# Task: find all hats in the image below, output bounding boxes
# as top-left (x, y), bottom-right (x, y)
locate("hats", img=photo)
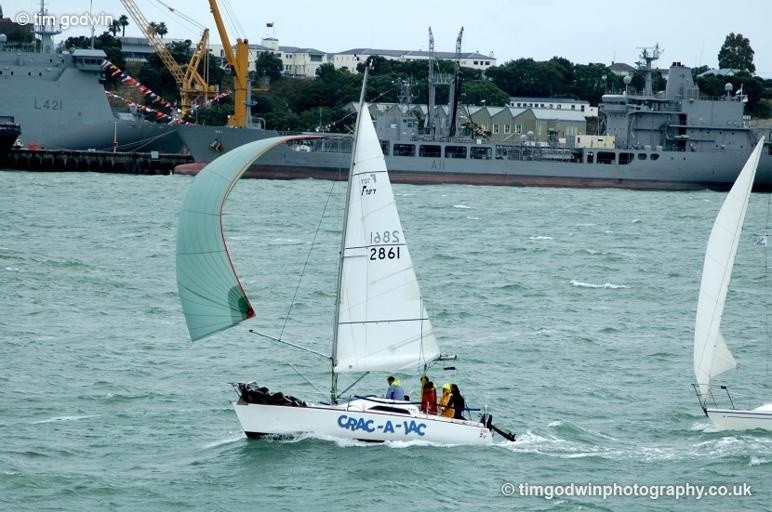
top-left (442, 384), bottom-right (450, 390)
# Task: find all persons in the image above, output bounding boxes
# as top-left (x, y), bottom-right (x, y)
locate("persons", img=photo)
top-left (419, 376), bottom-right (438, 415)
top-left (442, 384), bottom-right (464, 419)
top-left (386, 376), bottom-right (405, 400)
top-left (439, 382), bottom-right (455, 418)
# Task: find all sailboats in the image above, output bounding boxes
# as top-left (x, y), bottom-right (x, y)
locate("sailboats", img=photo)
top-left (173, 66), bottom-right (516, 449)
top-left (688, 137), bottom-right (771, 434)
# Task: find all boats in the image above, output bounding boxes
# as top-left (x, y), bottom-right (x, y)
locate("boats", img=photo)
top-left (172, 25), bottom-right (772, 195)
top-left (0, 0), bottom-right (191, 155)
